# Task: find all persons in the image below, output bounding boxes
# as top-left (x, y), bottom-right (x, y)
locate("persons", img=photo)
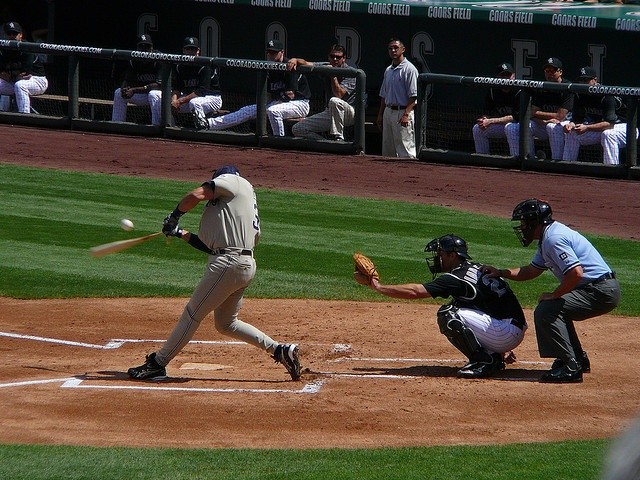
top-left (479, 198), bottom-right (620, 384)
top-left (529, 58), bottom-right (574, 161)
top-left (473, 63), bottom-right (521, 157)
top-left (192, 40), bottom-right (312, 136)
top-left (378, 38), bottom-right (419, 159)
top-left (562, 66), bottom-right (639, 165)
top-left (170, 37), bottom-right (223, 131)
top-left (112, 34), bottom-right (163, 127)
top-left (353, 234), bottom-right (528, 379)
top-left (292, 45), bottom-right (356, 141)
top-left (1, 23), bottom-right (49, 114)
top-left (129, 165), bottom-right (302, 381)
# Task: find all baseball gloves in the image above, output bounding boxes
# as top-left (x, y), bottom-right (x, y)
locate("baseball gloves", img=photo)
top-left (352, 252), bottom-right (379, 286)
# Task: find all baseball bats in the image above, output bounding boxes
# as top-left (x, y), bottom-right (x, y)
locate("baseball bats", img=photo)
top-left (88, 230), bottom-right (162, 256)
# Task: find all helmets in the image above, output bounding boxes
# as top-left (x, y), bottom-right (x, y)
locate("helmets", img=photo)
top-left (425, 234), bottom-right (472, 274)
top-left (211, 164), bottom-right (241, 178)
top-left (511, 198), bottom-right (552, 247)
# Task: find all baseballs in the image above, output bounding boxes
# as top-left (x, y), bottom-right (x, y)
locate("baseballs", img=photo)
top-left (120, 218), bottom-right (134, 232)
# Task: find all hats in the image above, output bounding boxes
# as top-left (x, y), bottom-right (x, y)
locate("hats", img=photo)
top-left (499, 63), bottom-right (516, 76)
top-left (266, 40), bottom-right (284, 52)
top-left (542, 57), bottom-right (563, 69)
top-left (184, 37), bottom-right (200, 49)
top-left (5, 22), bottom-right (22, 33)
top-left (577, 66), bottom-right (597, 79)
top-left (136, 33), bottom-right (153, 46)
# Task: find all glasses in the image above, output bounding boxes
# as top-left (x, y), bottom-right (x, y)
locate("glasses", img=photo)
top-left (329, 54), bottom-right (344, 60)
top-left (387, 45), bottom-right (402, 50)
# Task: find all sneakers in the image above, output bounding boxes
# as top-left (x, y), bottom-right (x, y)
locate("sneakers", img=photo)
top-left (191, 108), bottom-right (207, 132)
top-left (539, 360), bottom-right (584, 383)
top-left (271, 344), bottom-right (301, 382)
top-left (127, 352), bottom-right (167, 380)
top-left (578, 356), bottom-right (589, 372)
top-left (453, 355), bottom-right (498, 378)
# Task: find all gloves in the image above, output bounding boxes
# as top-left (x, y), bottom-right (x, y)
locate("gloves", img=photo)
top-left (169, 225), bottom-right (183, 240)
top-left (161, 210), bottom-right (183, 234)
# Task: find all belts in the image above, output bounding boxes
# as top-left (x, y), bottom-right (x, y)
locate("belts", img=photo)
top-left (591, 272), bottom-right (615, 283)
top-left (210, 249), bottom-right (254, 256)
top-left (500, 318), bottom-right (523, 331)
top-left (388, 104), bottom-right (408, 109)
